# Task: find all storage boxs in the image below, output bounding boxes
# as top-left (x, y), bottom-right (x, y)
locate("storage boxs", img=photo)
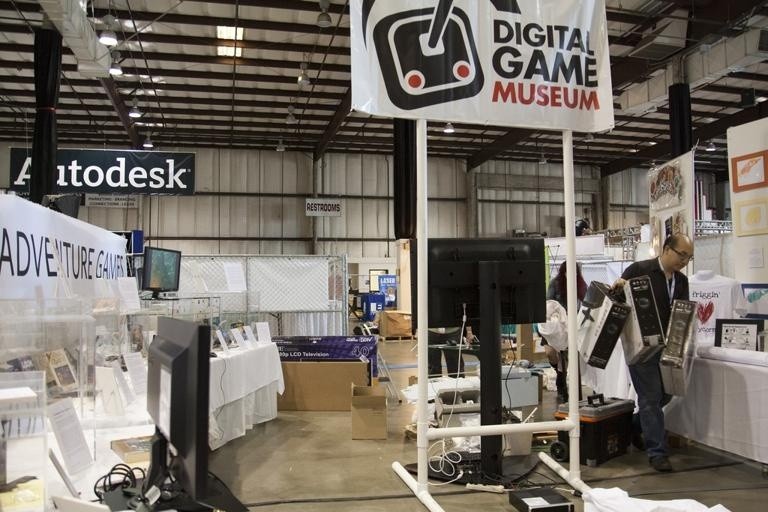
top-left (549, 392), bottom-right (636, 468)
top-left (350, 381), bottom-right (389, 441)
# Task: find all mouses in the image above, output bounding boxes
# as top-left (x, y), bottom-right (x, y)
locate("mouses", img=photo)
top-left (446, 339), bottom-right (457, 346)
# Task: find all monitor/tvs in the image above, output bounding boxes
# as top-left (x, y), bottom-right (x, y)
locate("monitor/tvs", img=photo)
top-left (126, 317), bottom-right (212, 510)
top-left (142, 247), bottom-right (181, 300)
top-left (410, 238), bottom-right (546, 328)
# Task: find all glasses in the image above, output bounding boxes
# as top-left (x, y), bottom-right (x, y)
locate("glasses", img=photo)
top-left (669, 246), bottom-right (694, 261)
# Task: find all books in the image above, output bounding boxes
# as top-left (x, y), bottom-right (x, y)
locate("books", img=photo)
top-left (109, 435), bottom-right (158, 464)
top-left (36, 347), bottom-right (79, 396)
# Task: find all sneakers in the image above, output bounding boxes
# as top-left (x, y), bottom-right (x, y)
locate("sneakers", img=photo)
top-left (631, 413), bottom-right (643, 449)
top-left (559, 394), bottom-right (568, 403)
top-left (649, 455), bottom-right (672, 471)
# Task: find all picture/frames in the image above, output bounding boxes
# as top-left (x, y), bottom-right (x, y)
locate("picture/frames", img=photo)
top-left (730, 149), bottom-right (766, 238)
top-left (713, 283), bottom-right (767, 351)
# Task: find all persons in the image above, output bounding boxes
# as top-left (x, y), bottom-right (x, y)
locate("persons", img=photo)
top-left (687, 268), bottom-right (749, 346)
top-left (540, 260), bottom-right (588, 403)
top-left (613, 232), bottom-right (695, 471)
top-left (426, 326), bottom-right (475, 377)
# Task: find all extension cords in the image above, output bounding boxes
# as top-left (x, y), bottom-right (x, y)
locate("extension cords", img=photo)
top-left (466, 483), bottom-right (505, 493)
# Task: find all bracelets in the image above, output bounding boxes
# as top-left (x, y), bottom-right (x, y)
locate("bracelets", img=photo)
top-left (466, 331), bottom-right (473, 333)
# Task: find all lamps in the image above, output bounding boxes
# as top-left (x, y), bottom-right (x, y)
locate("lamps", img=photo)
top-left (704, 137), bottom-right (716, 153)
top-left (442, 122), bottom-right (454, 134)
top-left (274, 0), bottom-right (333, 152)
top-left (97, 0), bottom-right (154, 149)
